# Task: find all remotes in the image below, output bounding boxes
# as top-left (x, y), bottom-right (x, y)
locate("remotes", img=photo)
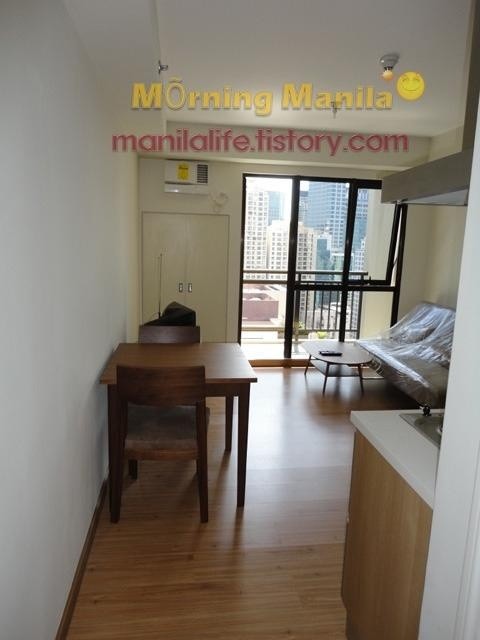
top-left (320, 350), bottom-right (342, 356)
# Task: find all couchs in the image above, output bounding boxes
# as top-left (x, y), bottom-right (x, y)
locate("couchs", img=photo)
top-left (353, 302), bottom-right (456, 409)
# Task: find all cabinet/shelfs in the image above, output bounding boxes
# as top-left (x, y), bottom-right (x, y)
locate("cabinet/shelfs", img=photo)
top-left (341, 429), bottom-right (433, 640)
top-left (141, 211), bottom-right (230, 343)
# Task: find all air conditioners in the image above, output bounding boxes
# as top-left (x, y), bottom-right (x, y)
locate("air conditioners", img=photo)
top-left (164, 160), bottom-right (209, 196)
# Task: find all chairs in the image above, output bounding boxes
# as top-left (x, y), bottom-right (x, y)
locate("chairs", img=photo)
top-left (138, 325), bottom-right (200, 344)
top-left (107, 364), bottom-right (210, 523)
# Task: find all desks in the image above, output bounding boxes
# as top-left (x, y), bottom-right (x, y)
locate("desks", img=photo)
top-left (100, 343), bottom-right (257, 507)
